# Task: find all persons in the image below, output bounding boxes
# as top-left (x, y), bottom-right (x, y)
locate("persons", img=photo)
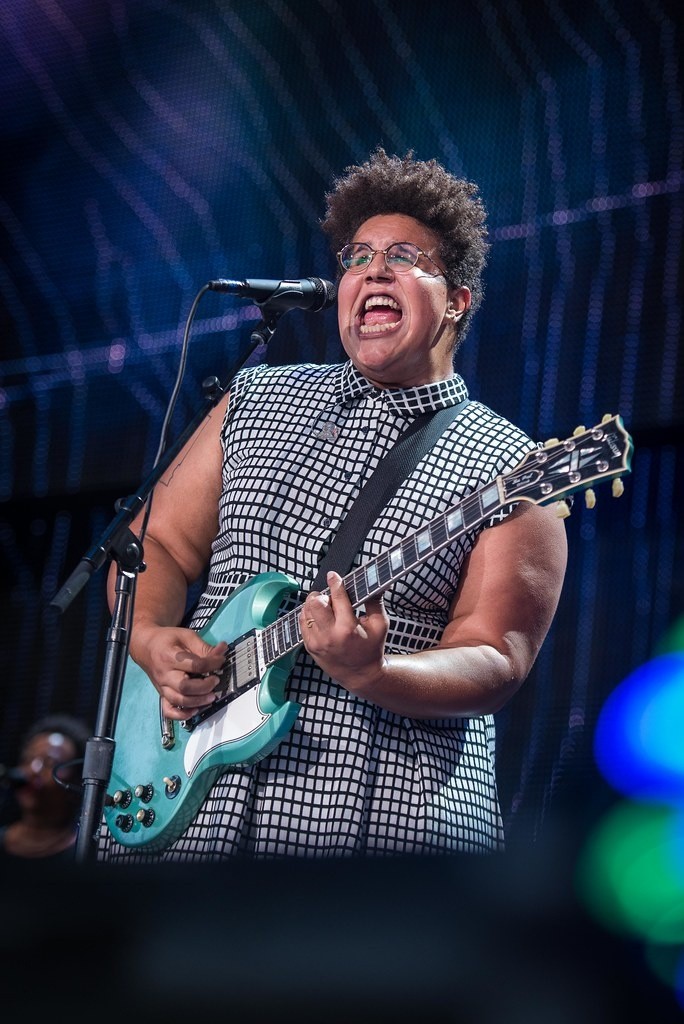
top-left (97, 153), bottom-right (566, 867)
top-left (0, 717), bottom-right (94, 876)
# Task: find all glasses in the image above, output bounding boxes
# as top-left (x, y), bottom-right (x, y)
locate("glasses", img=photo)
top-left (337, 240), bottom-right (456, 286)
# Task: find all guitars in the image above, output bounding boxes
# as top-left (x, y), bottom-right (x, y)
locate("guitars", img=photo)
top-left (97, 410), bottom-right (638, 859)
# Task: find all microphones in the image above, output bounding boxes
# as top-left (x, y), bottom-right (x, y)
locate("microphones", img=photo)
top-left (0, 768), bottom-right (28, 789)
top-left (207, 276), bottom-right (337, 313)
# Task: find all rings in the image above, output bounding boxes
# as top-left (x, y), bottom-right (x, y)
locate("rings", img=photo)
top-left (308, 619), bottom-right (315, 627)
